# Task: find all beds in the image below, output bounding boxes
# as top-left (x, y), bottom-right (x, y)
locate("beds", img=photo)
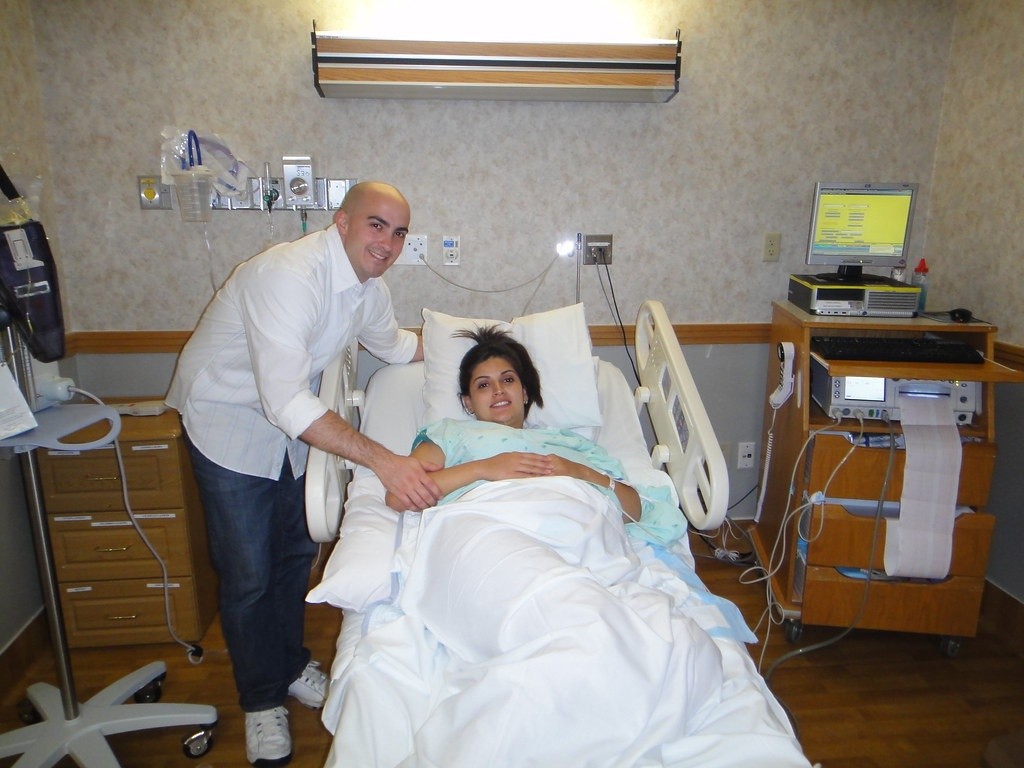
top-left (304, 300), bottom-right (814, 767)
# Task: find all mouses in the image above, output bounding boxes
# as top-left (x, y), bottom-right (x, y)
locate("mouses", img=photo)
top-left (950, 308), bottom-right (972, 324)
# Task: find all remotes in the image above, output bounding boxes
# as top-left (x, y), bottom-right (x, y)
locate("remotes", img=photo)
top-left (110, 400), bottom-right (168, 416)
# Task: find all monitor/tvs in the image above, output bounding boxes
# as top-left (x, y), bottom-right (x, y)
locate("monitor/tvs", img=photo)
top-left (804, 182), bottom-right (920, 281)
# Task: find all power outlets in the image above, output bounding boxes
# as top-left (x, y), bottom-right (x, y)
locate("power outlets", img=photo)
top-left (738, 442), bottom-right (754, 468)
top-left (395, 234), bottom-right (428, 266)
top-left (585, 234), bottom-right (612, 264)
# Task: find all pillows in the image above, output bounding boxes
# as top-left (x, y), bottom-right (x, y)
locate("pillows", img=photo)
top-left (417, 304), bottom-right (602, 428)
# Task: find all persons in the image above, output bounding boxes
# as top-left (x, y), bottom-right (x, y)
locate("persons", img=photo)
top-left (387, 327), bottom-right (642, 524)
top-left (170, 183), bottom-right (425, 760)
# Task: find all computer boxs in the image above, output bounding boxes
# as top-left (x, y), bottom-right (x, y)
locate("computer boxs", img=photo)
top-left (787, 275), bottom-right (921, 318)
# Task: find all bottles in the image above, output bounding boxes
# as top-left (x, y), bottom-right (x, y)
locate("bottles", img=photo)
top-left (911, 258), bottom-right (929, 312)
top-left (891, 266), bottom-right (906, 284)
top-left (172, 171), bottom-right (213, 223)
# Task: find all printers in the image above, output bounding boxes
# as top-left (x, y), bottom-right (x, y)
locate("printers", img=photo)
top-left (808, 358), bottom-right (982, 428)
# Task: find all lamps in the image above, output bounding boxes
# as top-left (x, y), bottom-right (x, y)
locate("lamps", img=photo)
top-left (309, 20), bottom-right (681, 102)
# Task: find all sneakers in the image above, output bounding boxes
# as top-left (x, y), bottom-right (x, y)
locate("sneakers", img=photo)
top-left (243, 705), bottom-right (292, 768)
top-left (286, 661), bottom-right (332, 710)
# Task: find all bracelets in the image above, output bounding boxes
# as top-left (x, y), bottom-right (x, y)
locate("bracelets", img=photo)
top-left (604, 474), bottom-right (615, 491)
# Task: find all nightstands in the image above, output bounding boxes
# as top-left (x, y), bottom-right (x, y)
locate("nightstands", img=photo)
top-left (37, 395), bottom-right (214, 644)
top-left (751, 302), bottom-right (1023, 639)
